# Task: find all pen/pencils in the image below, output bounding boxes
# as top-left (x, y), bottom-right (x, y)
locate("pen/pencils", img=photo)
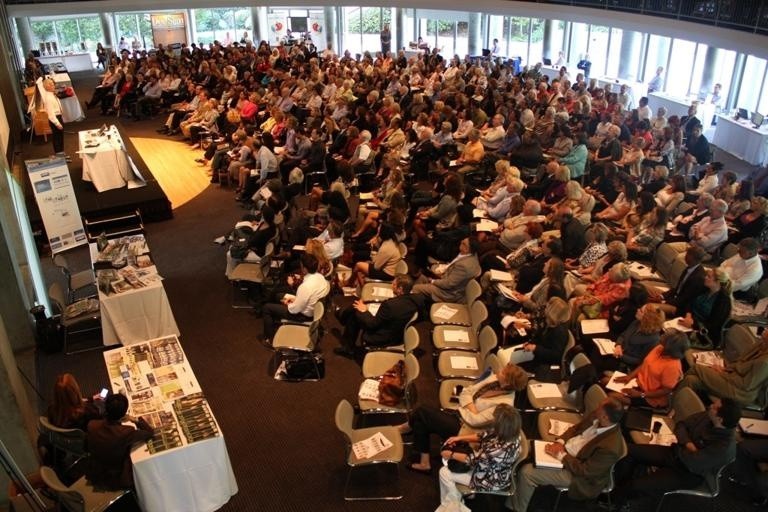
top-left (520, 307), bottom-right (523, 313)
top-left (525, 338), bottom-right (532, 346)
top-left (746, 424), bottom-right (754, 428)
top-left (555, 438), bottom-right (557, 443)
top-left (379, 438), bottom-right (384, 446)
top-left (616, 349), bottom-right (625, 358)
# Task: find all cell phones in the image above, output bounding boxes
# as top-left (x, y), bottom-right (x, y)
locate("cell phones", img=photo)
top-left (99, 387), bottom-right (109, 399)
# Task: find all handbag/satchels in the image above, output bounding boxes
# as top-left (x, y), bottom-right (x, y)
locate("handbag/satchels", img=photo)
top-left (378, 359), bottom-right (408, 407)
top-left (447, 441), bottom-right (474, 473)
top-left (267, 347), bottom-right (325, 382)
top-left (230, 234), bottom-right (250, 259)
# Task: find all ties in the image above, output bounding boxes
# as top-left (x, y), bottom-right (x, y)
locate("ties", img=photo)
top-left (54, 93), bottom-right (63, 114)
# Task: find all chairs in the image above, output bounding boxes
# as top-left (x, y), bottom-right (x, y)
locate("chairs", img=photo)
top-left (229, 195), bottom-right (768, 512)
top-left (49, 284), bottom-right (107, 357)
top-left (39, 415), bottom-right (94, 470)
top-left (52, 253), bottom-right (99, 300)
top-left (39, 464), bottom-right (138, 512)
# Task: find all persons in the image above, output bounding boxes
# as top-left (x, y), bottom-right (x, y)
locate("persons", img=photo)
top-left (43, 79), bottom-right (71, 159)
top-left (26, 53), bottom-right (45, 75)
top-left (47, 373), bottom-right (105, 453)
top-left (24, 61), bottom-right (41, 82)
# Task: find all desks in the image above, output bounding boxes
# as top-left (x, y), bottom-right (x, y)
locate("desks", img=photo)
top-left (87, 232), bottom-right (180, 348)
top-left (39, 62), bottom-right (80, 126)
top-left (102, 334), bottom-right (241, 512)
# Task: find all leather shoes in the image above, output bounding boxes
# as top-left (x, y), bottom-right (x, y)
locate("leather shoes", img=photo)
top-left (334, 306), bottom-right (345, 326)
top-left (333, 347), bottom-right (356, 361)
top-left (406, 462), bottom-right (432, 474)
top-left (65, 155), bottom-right (73, 163)
top-left (400, 429), bottom-right (413, 435)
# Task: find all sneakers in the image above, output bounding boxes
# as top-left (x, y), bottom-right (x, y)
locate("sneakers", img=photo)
top-left (84, 100), bottom-right (168, 121)
top-left (260, 339), bottom-right (273, 347)
top-left (156, 126), bottom-right (252, 210)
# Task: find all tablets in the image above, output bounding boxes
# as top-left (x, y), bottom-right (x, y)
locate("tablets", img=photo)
top-left (98, 124), bottom-right (105, 136)
